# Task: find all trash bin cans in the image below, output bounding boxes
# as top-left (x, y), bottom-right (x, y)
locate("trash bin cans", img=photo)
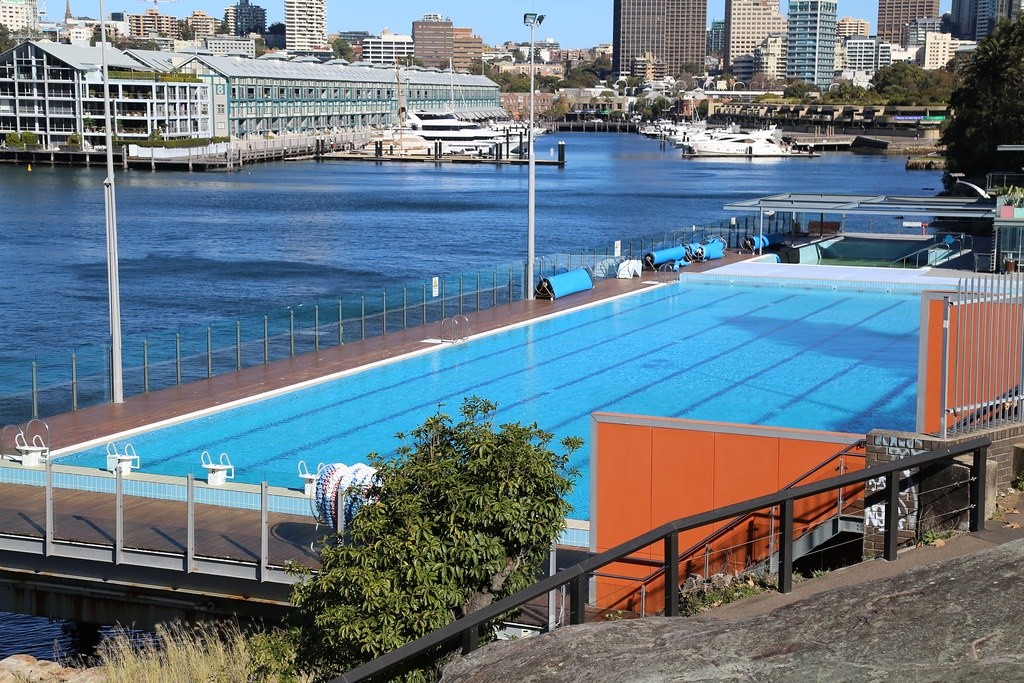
top-left (1005, 259), bottom-right (1015, 274)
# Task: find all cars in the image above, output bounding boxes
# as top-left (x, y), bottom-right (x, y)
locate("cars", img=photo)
top-left (593, 119), bottom-right (603, 123)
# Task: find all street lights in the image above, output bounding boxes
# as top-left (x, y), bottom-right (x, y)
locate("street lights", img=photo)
top-left (523, 12), bottom-right (548, 299)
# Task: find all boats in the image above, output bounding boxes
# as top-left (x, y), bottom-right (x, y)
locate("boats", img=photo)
top-left (667, 129), bottom-right (750, 147)
top-left (681, 133), bottom-right (821, 158)
top-left (508, 123), bottom-right (547, 134)
top-left (364, 127), bottom-right (451, 156)
top-left (640, 126), bottom-right (684, 136)
top-left (383, 47), bottom-right (538, 157)
